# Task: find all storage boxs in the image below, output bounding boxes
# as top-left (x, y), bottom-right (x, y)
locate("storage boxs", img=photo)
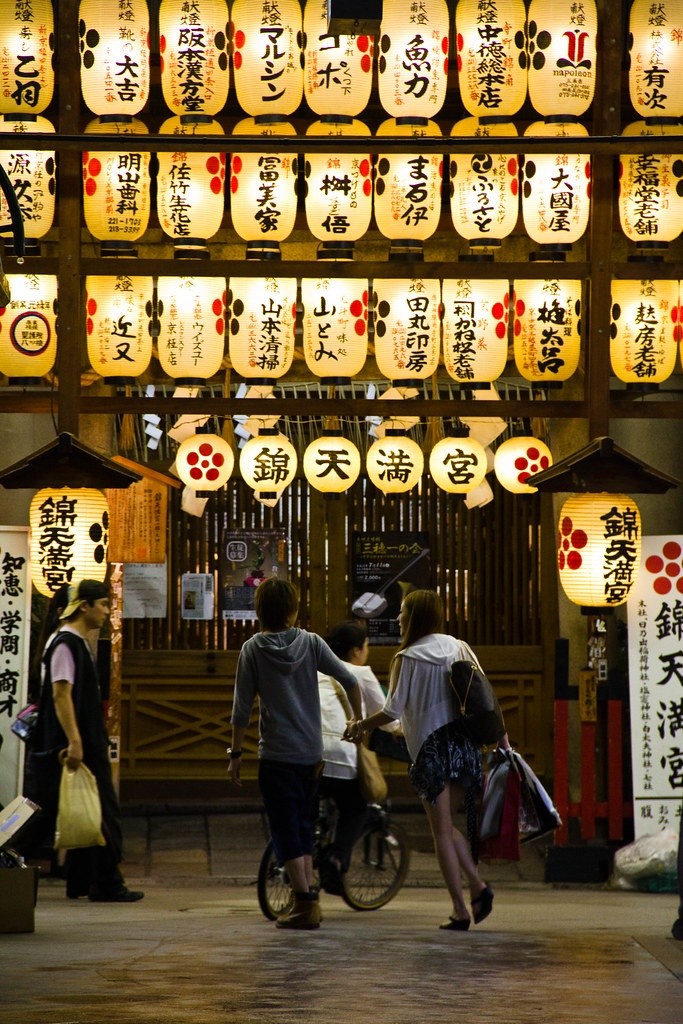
top-left (0, 795), bottom-right (42, 934)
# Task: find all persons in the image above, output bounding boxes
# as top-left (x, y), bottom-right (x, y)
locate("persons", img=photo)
top-left (9, 578), bottom-right (146, 905)
top-left (346, 588), bottom-right (511, 932)
top-left (226, 574), bottom-right (362, 931)
top-left (315, 617), bottom-right (405, 897)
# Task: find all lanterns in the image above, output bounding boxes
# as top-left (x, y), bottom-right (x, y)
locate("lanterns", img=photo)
top-left (0, 1), bottom-right (683, 392)
top-left (556, 491), bottom-right (642, 618)
top-left (26, 485), bottom-right (111, 610)
top-left (175, 426), bottom-right (555, 500)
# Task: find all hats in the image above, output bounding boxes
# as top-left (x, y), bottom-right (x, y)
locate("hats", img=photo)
top-left (59, 578), bottom-right (108, 620)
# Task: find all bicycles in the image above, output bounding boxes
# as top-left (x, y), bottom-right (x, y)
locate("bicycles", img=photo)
top-left (250, 792), bottom-right (413, 921)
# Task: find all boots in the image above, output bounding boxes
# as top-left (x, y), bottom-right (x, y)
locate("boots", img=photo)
top-left (277, 889), bottom-right (320, 930)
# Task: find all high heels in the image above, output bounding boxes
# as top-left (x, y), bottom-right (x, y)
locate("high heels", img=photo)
top-left (471, 887), bottom-right (494, 924)
top-left (439, 916), bottom-right (470, 931)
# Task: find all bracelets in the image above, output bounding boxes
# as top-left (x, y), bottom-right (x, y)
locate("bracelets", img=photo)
top-left (225, 747), bottom-right (243, 760)
top-left (356, 719), bottom-right (368, 735)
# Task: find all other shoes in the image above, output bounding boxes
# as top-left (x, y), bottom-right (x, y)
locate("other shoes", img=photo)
top-left (66, 886), bottom-right (91, 899)
top-left (322, 860), bottom-right (342, 896)
top-left (89, 885), bottom-right (144, 903)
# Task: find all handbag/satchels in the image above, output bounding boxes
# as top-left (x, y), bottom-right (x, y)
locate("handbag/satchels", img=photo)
top-left (473, 748), bottom-right (563, 862)
top-left (358, 745), bottom-right (387, 803)
top-left (449, 640), bottom-right (504, 739)
top-left (11, 703), bottom-right (40, 741)
top-left (53, 758), bottom-right (106, 849)
